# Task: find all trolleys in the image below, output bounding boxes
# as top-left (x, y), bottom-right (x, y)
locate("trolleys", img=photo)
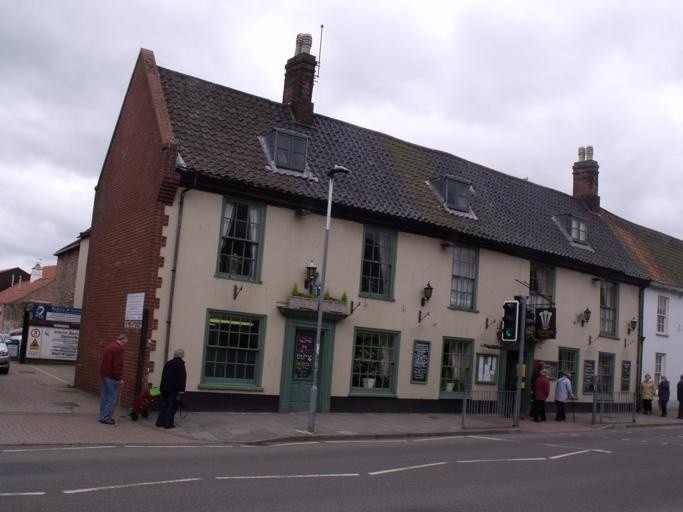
top-left (129, 382), bottom-right (166, 425)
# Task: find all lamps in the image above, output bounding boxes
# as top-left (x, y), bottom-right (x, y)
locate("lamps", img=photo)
top-left (578, 306), bottom-right (593, 326)
top-left (625, 314), bottom-right (638, 335)
top-left (419, 281), bottom-right (434, 307)
top-left (302, 257), bottom-right (320, 290)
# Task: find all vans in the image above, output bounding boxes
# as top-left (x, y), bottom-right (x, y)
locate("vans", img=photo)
top-left (0, 333), bottom-right (11, 374)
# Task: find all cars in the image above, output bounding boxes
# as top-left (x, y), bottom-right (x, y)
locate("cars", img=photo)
top-left (2, 327), bottom-right (22, 358)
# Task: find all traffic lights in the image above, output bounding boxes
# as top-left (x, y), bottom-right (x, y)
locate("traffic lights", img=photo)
top-left (498, 299), bottom-right (518, 341)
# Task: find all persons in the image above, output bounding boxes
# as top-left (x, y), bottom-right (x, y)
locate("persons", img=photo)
top-left (676, 374), bottom-right (683, 419)
top-left (528, 362), bottom-right (549, 414)
top-left (156, 348), bottom-right (186, 428)
top-left (659, 376), bottom-right (670, 417)
top-left (532, 369), bottom-right (550, 423)
top-left (554, 372), bottom-right (578, 422)
top-left (639, 374), bottom-right (656, 415)
top-left (97, 334), bottom-right (128, 425)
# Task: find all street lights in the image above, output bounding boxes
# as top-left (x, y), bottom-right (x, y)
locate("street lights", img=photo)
top-left (306, 157), bottom-right (351, 436)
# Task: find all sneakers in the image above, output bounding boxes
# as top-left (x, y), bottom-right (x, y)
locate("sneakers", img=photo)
top-left (98, 416), bottom-right (116, 425)
